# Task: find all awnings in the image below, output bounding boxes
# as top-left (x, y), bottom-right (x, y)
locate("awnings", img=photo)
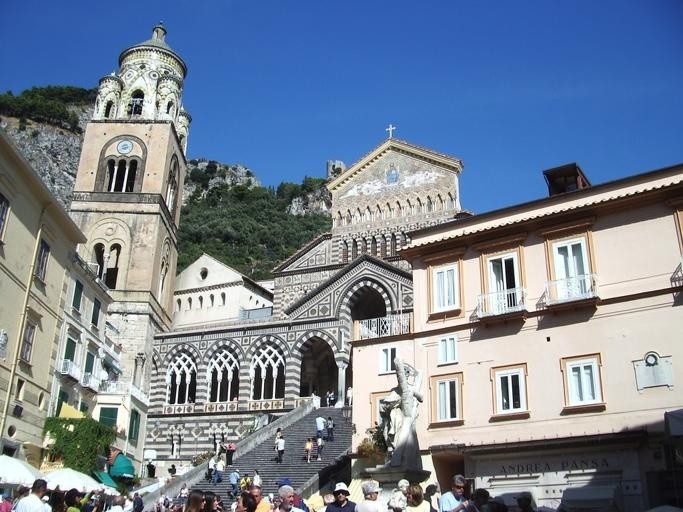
top-left (93, 471), bottom-right (118, 488)
top-left (109, 454), bottom-right (134, 479)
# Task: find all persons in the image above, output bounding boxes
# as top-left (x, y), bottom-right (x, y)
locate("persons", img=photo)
top-left (0, 474), bottom-right (536, 512)
top-left (326, 417), bottom-right (335, 441)
top-left (147, 460), bottom-right (156, 478)
top-left (304, 438), bottom-right (313, 464)
top-left (316, 414), bottom-right (327, 439)
top-left (385, 358), bottom-right (425, 465)
top-left (274, 428), bottom-right (281, 451)
top-left (326, 389), bottom-right (335, 407)
top-left (168, 464), bottom-right (176, 478)
top-left (275, 436), bottom-right (285, 464)
top-left (316, 437), bottom-right (325, 461)
top-left (346, 387), bottom-right (352, 406)
top-left (207, 455), bottom-right (260, 497)
top-left (225, 441), bottom-right (237, 468)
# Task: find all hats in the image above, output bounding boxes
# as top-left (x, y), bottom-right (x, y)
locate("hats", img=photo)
top-left (333, 481), bottom-right (350, 496)
top-left (360, 478), bottom-right (381, 495)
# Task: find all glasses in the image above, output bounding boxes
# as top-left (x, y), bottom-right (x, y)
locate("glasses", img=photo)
top-left (453, 484), bottom-right (465, 490)
